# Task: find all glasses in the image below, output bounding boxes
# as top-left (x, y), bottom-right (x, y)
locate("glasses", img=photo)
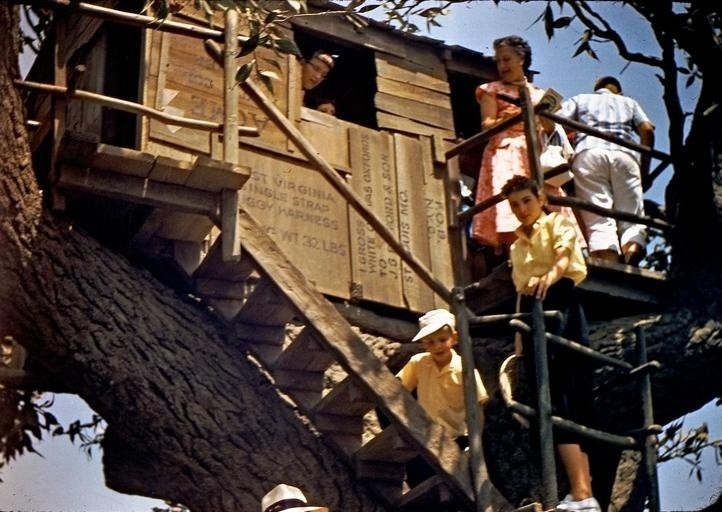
top-left (307, 61), bottom-right (328, 78)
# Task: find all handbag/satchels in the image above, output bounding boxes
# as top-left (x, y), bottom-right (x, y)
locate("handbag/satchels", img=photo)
top-left (540, 145), bottom-right (574, 188)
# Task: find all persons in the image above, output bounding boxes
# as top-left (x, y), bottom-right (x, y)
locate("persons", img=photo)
top-left (315, 97), bottom-right (336, 117)
top-left (396, 309), bottom-right (490, 500)
top-left (261, 483), bottom-right (328, 512)
top-left (476, 35), bottom-right (562, 256)
top-left (499, 174), bottom-right (602, 512)
top-left (301, 50), bottom-right (335, 106)
top-left (539, 76), bottom-right (655, 263)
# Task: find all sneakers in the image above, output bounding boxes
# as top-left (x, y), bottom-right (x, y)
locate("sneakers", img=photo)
top-left (556, 494), bottom-right (602, 512)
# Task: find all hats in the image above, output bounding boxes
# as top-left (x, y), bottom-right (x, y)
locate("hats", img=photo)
top-left (261, 483), bottom-right (329, 512)
top-left (411, 308), bottom-right (456, 343)
